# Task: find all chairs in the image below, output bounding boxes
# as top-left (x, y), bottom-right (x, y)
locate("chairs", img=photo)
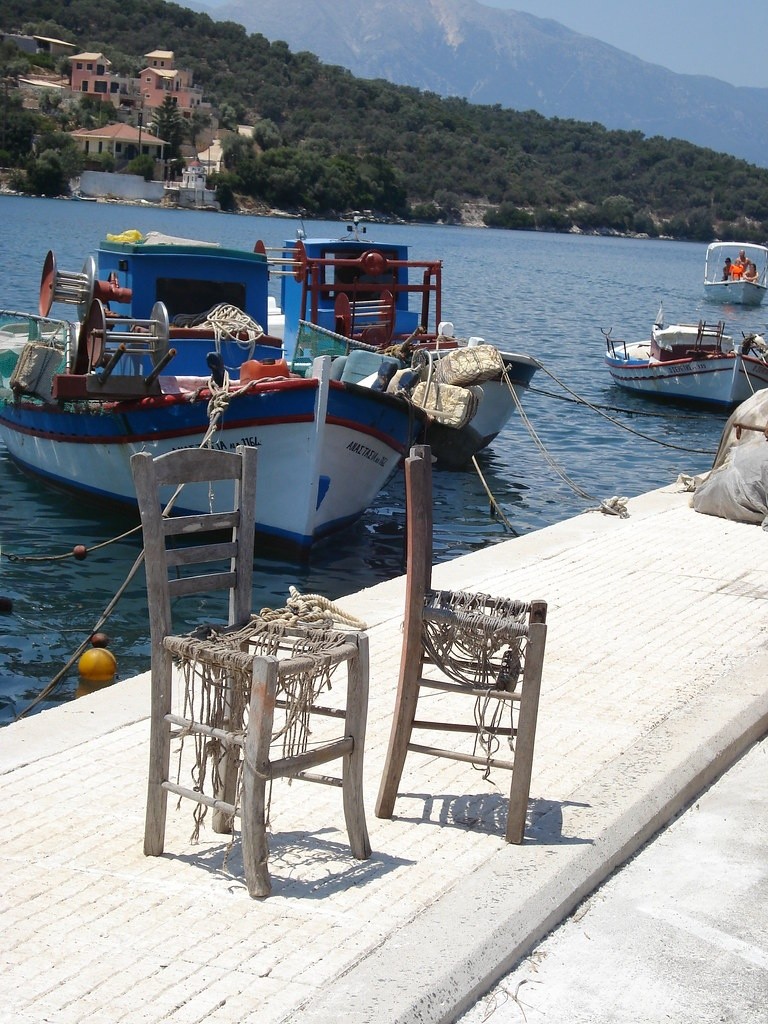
top-left (373, 444), bottom-right (549, 847)
top-left (129, 444), bottom-right (368, 898)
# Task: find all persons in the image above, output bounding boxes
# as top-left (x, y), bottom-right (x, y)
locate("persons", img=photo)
top-left (734, 250), bottom-right (750, 279)
top-left (743, 263), bottom-right (760, 283)
top-left (722, 256), bottom-right (733, 280)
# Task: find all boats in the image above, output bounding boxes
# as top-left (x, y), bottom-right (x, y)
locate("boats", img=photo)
top-left (1, 231), bottom-right (432, 562)
top-left (267, 209), bottom-right (547, 475)
top-left (702, 241), bottom-right (768, 306)
top-left (598, 299), bottom-right (768, 411)
